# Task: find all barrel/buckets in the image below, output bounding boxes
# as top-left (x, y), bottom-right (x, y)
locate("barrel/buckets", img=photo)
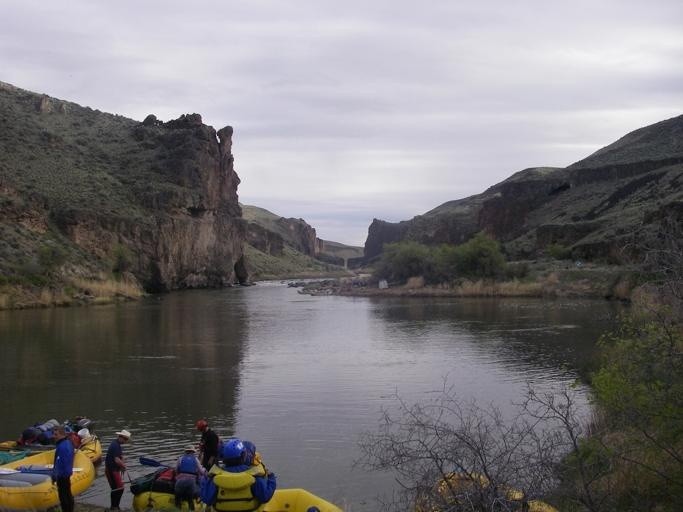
top-left (77, 428), bottom-right (91, 438)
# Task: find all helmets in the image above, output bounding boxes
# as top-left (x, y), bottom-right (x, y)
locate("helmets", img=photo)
top-left (224, 439), bottom-right (245, 458)
top-left (197, 420), bottom-right (208, 430)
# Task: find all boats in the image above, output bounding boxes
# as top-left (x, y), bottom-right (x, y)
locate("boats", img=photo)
top-left (252, 487), bottom-right (342, 512)
top-left (0, 432), bottom-right (101, 509)
top-left (417, 470), bottom-right (557, 512)
top-left (132, 449), bottom-right (266, 512)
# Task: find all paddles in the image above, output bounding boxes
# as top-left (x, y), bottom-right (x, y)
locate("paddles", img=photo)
top-left (139, 457), bottom-right (171, 468)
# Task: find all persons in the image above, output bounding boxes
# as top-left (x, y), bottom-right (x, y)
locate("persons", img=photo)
top-left (174, 445), bottom-right (206, 512)
top-left (196, 419), bottom-right (219, 470)
top-left (105, 430), bottom-right (131, 511)
top-left (51, 426), bottom-right (74, 512)
top-left (201, 440), bottom-right (276, 512)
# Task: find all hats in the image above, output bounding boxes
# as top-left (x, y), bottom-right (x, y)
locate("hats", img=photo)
top-left (115, 430), bottom-right (131, 438)
top-left (184, 445), bottom-right (197, 453)
